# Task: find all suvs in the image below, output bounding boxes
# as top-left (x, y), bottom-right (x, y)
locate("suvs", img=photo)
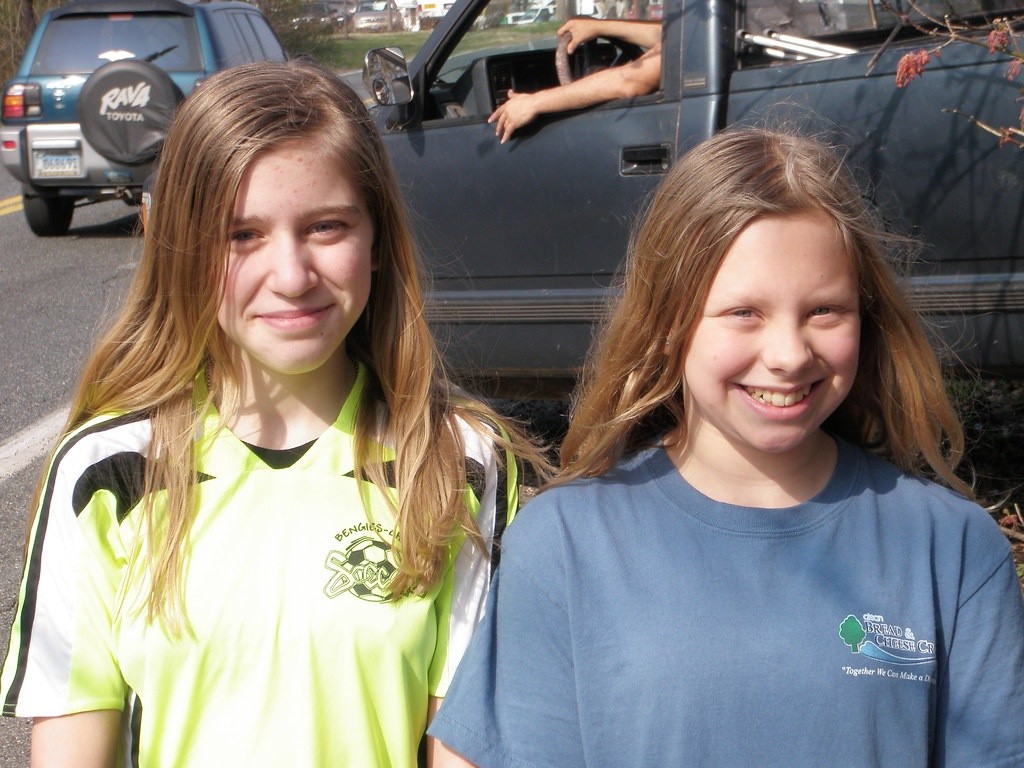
top-left (2, 0), bottom-right (284, 238)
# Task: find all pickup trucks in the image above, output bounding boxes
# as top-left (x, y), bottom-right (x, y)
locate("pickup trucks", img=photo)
top-left (358, 3), bottom-right (1024, 455)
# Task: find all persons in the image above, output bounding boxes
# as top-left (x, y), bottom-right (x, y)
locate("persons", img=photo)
top-left (0, 64), bottom-right (558, 768)
top-left (486, 13), bottom-right (662, 144)
top-left (424, 125), bottom-right (1024, 768)
